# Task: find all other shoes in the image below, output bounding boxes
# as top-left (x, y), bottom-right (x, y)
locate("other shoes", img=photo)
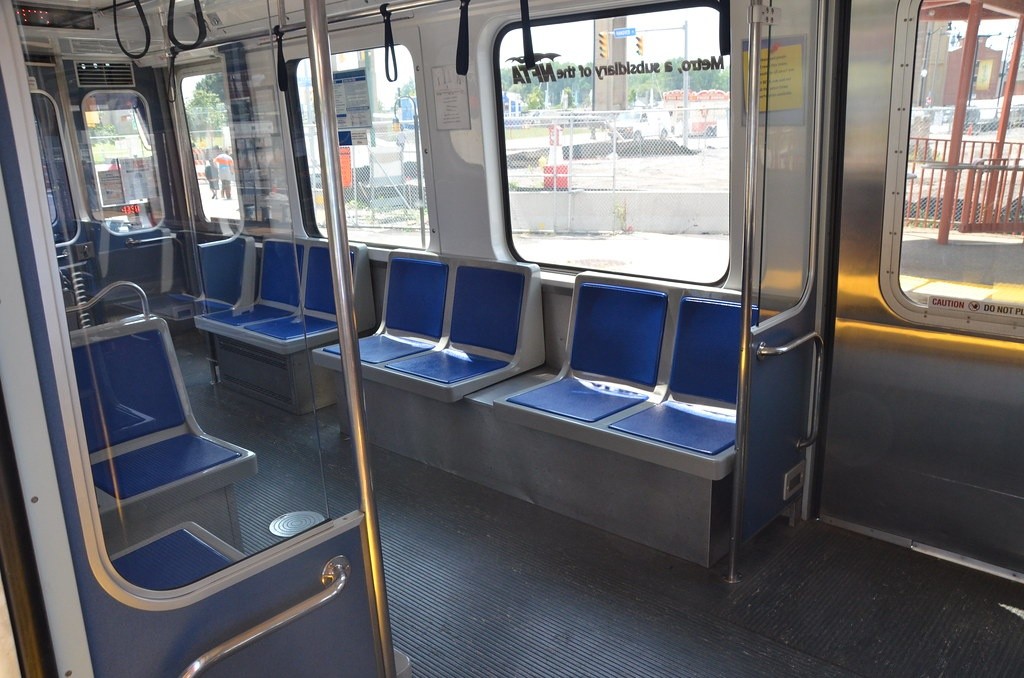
top-left (212, 194), bottom-right (216, 199)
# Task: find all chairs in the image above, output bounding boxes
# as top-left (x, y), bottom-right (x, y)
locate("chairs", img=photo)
top-left (68, 311), bottom-right (259, 552)
top-left (195, 233), bottom-right (766, 567)
top-left (103, 522), bottom-right (414, 677)
top-left (55, 220), bottom-right (181, 328)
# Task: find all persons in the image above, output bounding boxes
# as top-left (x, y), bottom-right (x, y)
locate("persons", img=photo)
top-left (215, 151), bottom-right (234, 197)
top-left (206, 158), bottom-right (220, 198)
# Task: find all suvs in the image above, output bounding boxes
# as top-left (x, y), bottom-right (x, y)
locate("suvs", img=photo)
top-left (610, 110), bottom-right (667, 140)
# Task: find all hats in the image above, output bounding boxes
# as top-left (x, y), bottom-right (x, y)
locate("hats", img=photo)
top-left (209, 158), bottom-right (213, 162)
